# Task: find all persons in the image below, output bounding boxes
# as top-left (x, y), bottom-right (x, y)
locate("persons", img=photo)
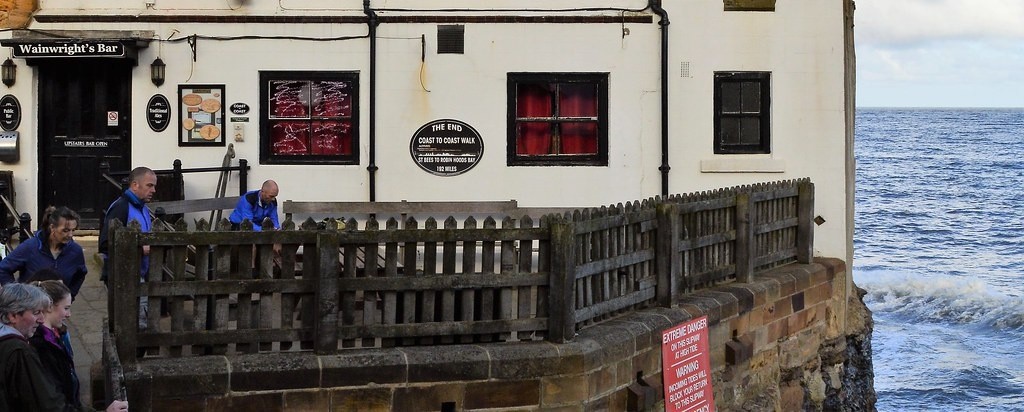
top-left (28, 281), bottom-right (80, 411)
top-left (229, 180), bottom-right (282, 253)
top-left (1, 206), bottom-right (88, 304)
top-left (0, 284), bottom-right (129, 412)
top-left (98, 167), bottom-right (157, 357)
